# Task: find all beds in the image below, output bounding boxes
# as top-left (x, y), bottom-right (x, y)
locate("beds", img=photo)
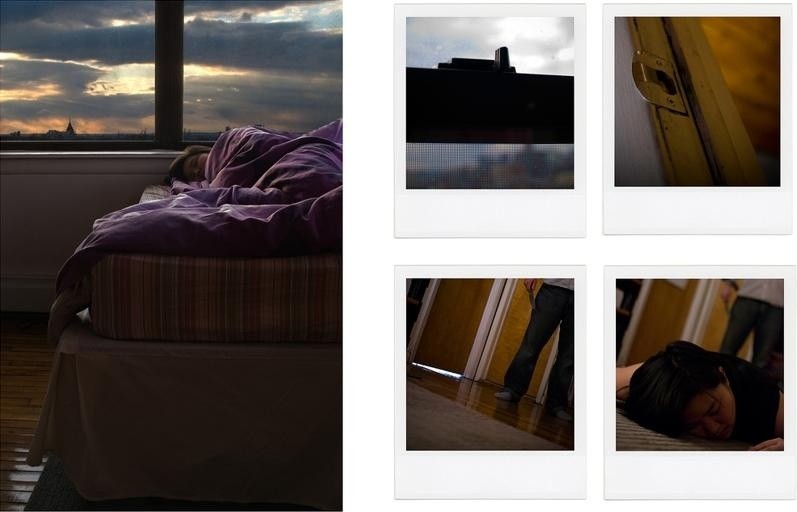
top-left (25, 117), bottom-right (343, 511)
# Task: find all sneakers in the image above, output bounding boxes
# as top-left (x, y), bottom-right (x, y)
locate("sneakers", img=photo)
top-left (494, 388), bottom-right (520, 402)
top-left (553, 407), bottom-right (573, 421)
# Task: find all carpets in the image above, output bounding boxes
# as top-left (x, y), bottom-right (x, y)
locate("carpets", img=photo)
top-left (26, 458), bottom-right (322, 512)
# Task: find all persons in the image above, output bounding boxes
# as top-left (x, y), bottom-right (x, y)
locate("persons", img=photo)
top-left (615, 336), bottom-right (783, 452)
top-left (166, 141), bottom-right (212, 183)
top-left (490, 277), bottom-right (575, 423)
top-left (717, 279), bottom-right (783, 372)
